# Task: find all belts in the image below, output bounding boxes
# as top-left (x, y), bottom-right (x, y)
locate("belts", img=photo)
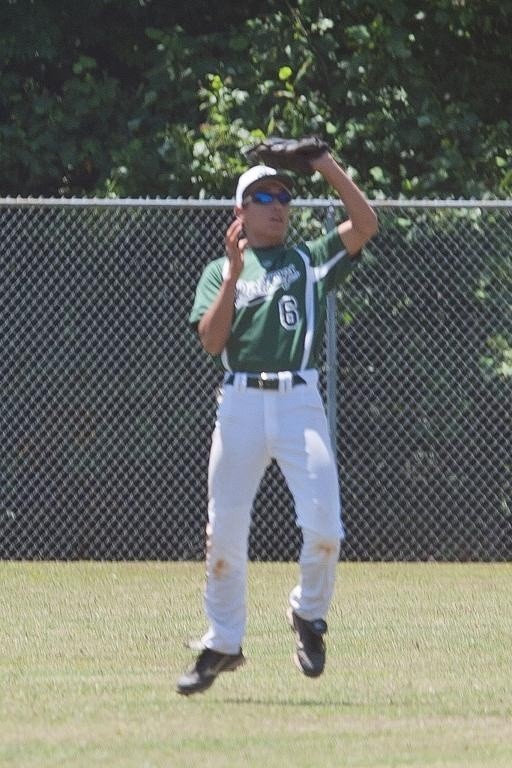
top-left (226, 374), bottom-right (306, 388)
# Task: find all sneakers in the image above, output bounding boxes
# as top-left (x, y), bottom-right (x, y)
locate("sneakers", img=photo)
top-left (285, 607), bottom-right (328, 676)
top-left (173, 646), bottom-right (247, 694)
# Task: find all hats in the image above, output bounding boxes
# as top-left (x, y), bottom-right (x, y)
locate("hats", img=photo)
top-left (235, 164), bottom-right (295, 210)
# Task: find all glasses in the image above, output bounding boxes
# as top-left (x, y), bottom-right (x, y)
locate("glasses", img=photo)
top-left (243, 191), bottom-right (292, 206)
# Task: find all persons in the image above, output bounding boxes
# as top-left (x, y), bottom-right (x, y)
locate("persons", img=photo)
top-left (176, 134), bottom-right (382, 697)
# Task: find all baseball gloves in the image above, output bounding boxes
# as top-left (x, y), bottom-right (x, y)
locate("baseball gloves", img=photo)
top-left (245, 133), bottom-right (330, 177)
